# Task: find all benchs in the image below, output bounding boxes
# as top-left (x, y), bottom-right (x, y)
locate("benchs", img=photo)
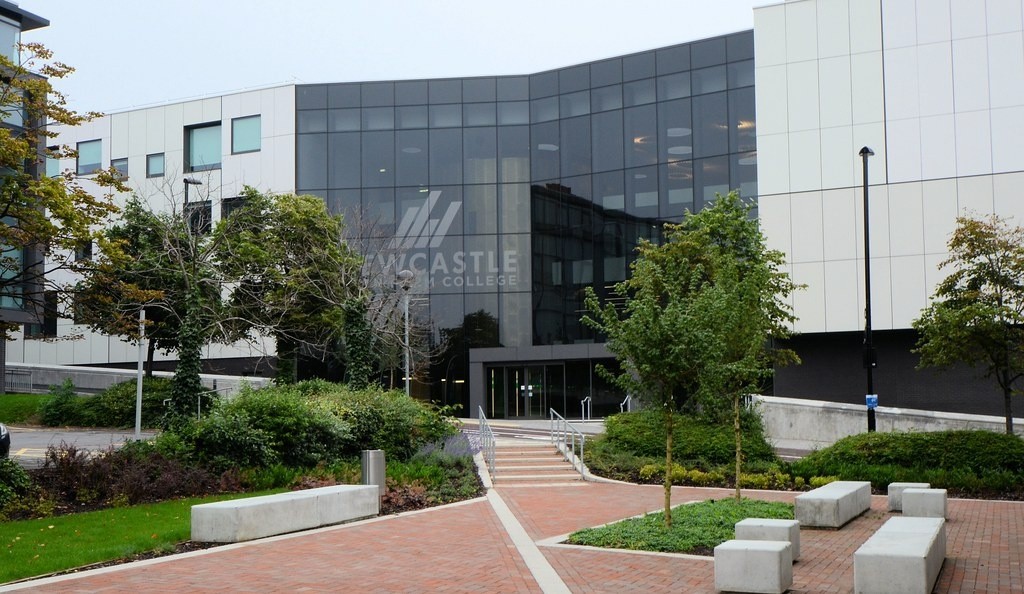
top-left (713, 481), bottom-right (947, 594)
top-left (190, 485), bottom-right (379, 542)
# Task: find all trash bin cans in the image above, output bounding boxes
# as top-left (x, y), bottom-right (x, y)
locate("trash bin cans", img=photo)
top-left (361, 449), bottom-right (387, 496)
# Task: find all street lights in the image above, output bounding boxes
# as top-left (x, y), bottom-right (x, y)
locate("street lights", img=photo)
top-left (859, 145), bottom-right (878, 430)
top-left (396, 269), bottom-right (415, 398)
top-left (184, 178), bottom-right (202, 231)
